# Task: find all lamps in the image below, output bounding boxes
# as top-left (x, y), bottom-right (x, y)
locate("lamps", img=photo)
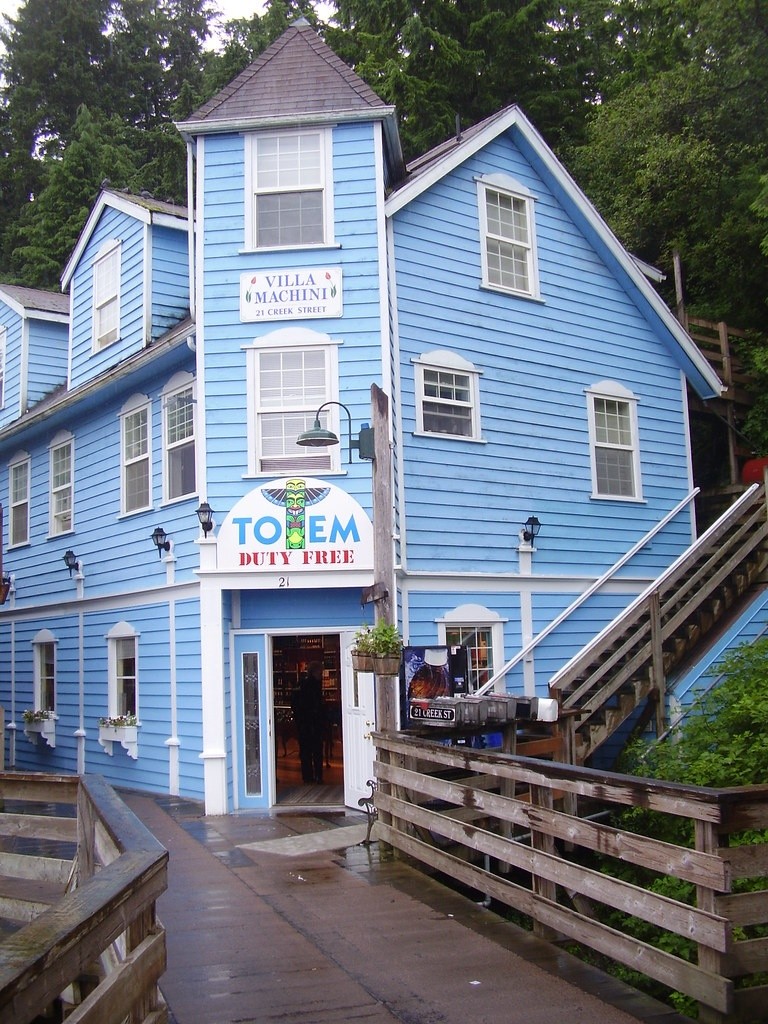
top-left (0, 574), bottom-right (11, 605)
top-left (150, 528), bottom-right (167, 558)
top-left (62, 549), bottom-right (78, 578)
top-left (195, 504), bottom-right (213, 539)
top-left (523, 517), bottom-right (541, 548)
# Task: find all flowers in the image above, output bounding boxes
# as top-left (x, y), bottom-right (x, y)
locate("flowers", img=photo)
top-left (99, 716), bottom-right (136, 727)
top-left (21, 711), bottom-right (49, 724)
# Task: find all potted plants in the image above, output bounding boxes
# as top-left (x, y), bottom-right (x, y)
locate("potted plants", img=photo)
top-left (348, 625), bottom-right (376, 673)
top-left (370, 621), bottom-right (403, 677)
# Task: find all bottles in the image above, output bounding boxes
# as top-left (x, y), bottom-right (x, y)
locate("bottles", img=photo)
top-left (299, 638), bottom-right (322, 649)
top-left (275, 676), bottom-right (295, 724)
top-left (408, 649), bottom-right (455, 727)
top-left (320, 651), bottom-right (337, 702)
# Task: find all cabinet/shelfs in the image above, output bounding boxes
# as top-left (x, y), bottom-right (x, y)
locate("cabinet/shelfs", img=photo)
top-left (274, 651), bottom-right (337, 705)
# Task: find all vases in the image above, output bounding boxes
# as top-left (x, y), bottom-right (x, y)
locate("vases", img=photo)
top-left (99, 726), bottom-right (137, 760)
top-left (23, 720), bottom-right (55, 749)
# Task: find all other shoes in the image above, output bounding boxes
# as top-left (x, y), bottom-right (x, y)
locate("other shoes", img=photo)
top-left (315, 775), bottom-right (323, 784)
top-left (304, 775), bottom-right (314, 783)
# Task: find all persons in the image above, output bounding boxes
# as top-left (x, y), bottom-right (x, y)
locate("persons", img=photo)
top-left (291, 662), bottom-right (325, 785)
